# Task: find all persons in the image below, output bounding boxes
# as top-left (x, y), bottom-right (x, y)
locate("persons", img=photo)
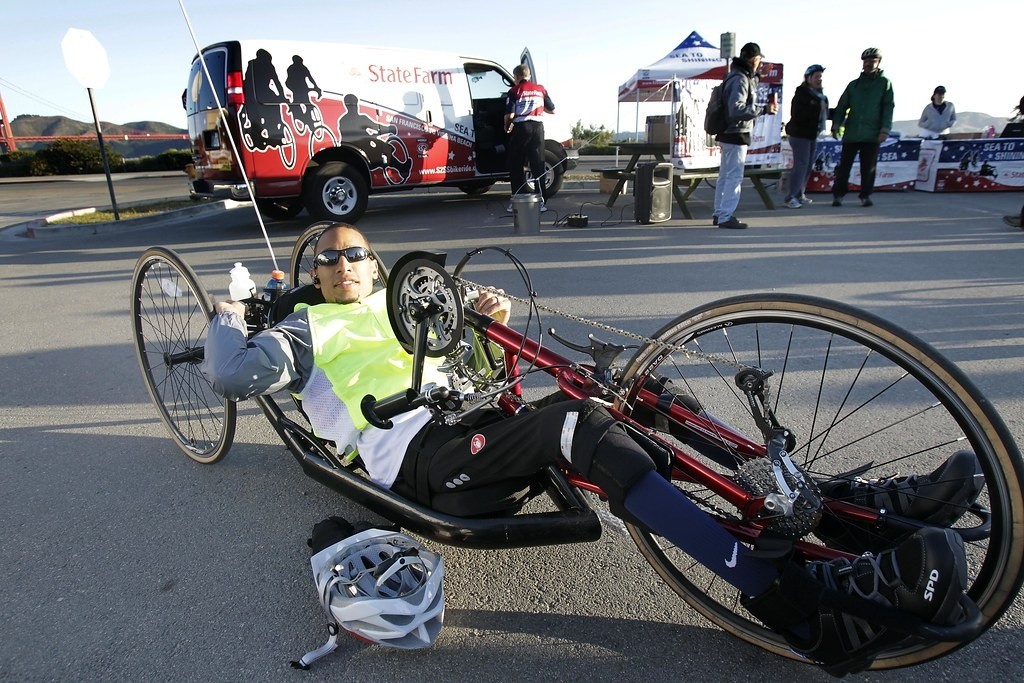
top-left (502, 64), bottom-right (555, 212)
top-left (713, 42), bottom-right (778, 228)
top-left (830, 47), bottom-right (895, 207)
top-left (918, 85), bottom-right (957, 140)
top-left (203, 220), bottom-right (984, 680)
top-left (781, 64), bottom-right (829, 209)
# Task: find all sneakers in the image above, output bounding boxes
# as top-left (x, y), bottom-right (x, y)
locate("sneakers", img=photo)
top-left (784, 198), bottom-right (802, 209)
top-left (799, 194), bottom-right (813, 204)
top-left (713, 216), bottom-right (719, 226)
top-left (742, 526), bottom-right (968, 678)
top-left (719, 216), bottom-right (747, 229)
top-left (813, 451), bottom-right (986, 555)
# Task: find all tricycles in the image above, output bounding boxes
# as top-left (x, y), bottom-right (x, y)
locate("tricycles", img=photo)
top-left (128, 217), bottom-right (1023, 670)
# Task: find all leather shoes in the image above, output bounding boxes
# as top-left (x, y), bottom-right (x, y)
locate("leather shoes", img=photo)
top-left (1003, 215), bottom-right (1024, 228)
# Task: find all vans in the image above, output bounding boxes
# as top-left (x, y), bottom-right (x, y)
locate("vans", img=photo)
top-left (181, 38), bottom-right (571, 227)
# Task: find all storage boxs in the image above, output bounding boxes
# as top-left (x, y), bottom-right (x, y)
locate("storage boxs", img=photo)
top-left (599, 173), bottom-right (628, 194)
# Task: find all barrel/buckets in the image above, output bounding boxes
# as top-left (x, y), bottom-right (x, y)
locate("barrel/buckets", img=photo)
top-left (510, 180), bottom-right (543, 235)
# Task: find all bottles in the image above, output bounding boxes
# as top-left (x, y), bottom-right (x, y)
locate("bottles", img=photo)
top-left (767, 92), bottom-right (778, 115)
top-left (228, 262), bottom-right (257, 301)
top-left (263, 269), bottom-right (288, 302)
top-left (981, 125), bottom-right (995, 139)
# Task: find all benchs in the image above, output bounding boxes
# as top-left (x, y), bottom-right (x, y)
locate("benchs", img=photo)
top-left (591, 167), bottom-right (781, 219)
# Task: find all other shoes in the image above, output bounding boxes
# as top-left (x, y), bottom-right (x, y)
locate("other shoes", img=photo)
top-left (832, 196), bottom-right (843, 205)
top-left (507, 203), bottom-right (514, 212)
top-left (861, 198), bottom-right (872, 206)
top-left (540, 202), bottom-right (547, 212)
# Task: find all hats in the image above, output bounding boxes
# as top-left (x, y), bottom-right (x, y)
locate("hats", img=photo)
top-left (804, 65), bottom-right (825, 77)
top-left (741, 42), bottom-right (764, 58)
top-left (931, 86), bottom-right (946, 101)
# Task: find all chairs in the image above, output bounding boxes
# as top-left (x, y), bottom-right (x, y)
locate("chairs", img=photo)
top-left (475, 110), bottom-right (496, 150)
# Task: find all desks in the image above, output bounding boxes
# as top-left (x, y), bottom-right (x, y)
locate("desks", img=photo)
top-left (607, 141), bottom-right (774, 219)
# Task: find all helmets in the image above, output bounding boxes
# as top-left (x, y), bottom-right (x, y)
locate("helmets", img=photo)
top-left (299, 529), bottom-right (445, 666)
top-left (861, 48), bottom-right (881, 60)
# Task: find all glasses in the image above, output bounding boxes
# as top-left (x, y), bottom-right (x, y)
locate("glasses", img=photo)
top-left (313, 247), bottom-right (373, 269)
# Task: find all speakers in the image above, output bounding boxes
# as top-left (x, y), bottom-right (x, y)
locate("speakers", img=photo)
top-left (634, 162), bottom-right (673, 224)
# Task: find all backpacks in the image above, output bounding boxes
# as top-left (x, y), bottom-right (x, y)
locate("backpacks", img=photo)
top-left (704, 75), bottom-right (750, 135)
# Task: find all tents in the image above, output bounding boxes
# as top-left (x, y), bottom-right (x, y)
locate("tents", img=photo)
top-left (615, 32), bottom-right (783, 167)
